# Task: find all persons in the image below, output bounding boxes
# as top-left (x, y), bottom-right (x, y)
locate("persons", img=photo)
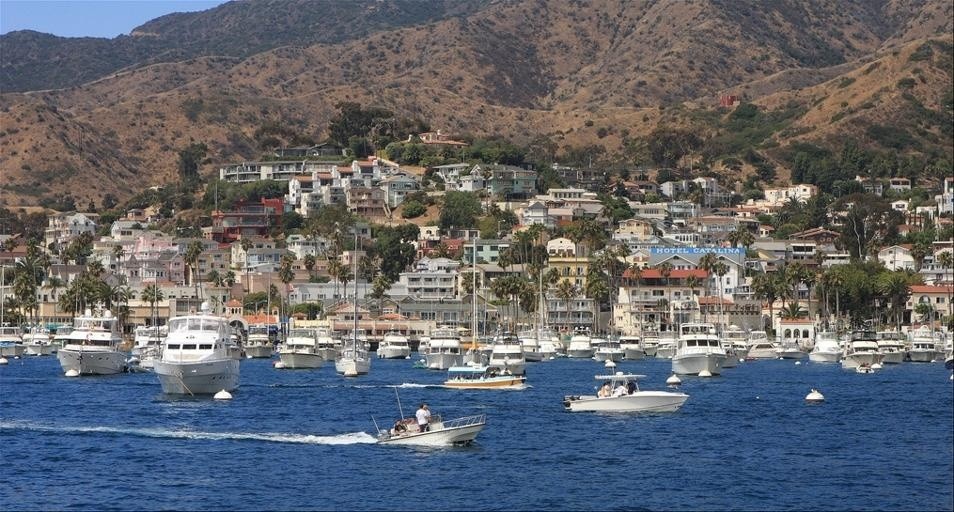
top-left (415, 403), bottom-right (430, 432)
top-left (421, 405), bottom-right (432, 430)
top-left (598, 379), bottom-right (636, 396)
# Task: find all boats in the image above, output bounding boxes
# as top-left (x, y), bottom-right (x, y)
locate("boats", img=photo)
top-left (242, 273), bottom-right (338, 369)
top-left (376, 301), bottom-right (410, 359)
top-left (370, 386), bottom-right (488, 448)
top-left (1, 277), bottom-right (168, 376)
top-left (153, 269), bottom-right (241, 394)
top-left (560, 372), bottom-right (691, 414)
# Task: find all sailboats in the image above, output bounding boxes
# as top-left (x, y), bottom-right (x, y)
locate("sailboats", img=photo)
top-left (335, 227), bottom-right (371, 374)
top-left (418, 237), bottom-right (954, 389)
